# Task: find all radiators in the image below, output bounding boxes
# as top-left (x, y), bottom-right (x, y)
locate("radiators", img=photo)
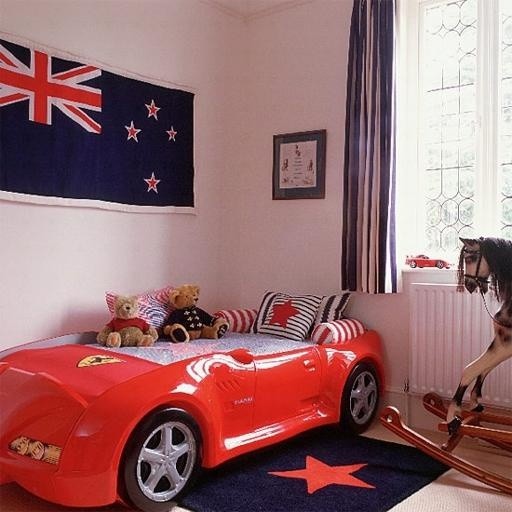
top-left (406, 280), bottom-right (510, 412)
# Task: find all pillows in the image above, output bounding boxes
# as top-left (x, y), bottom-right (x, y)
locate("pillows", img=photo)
top-left (213, 289), bottom-right (365, 347)
top-left (103, 284), bottom-right (174, 340)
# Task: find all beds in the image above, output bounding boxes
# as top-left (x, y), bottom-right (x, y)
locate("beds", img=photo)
top-left (1, 327), bottom-right (384, 509)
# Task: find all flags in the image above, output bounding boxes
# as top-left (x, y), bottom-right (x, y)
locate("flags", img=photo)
top-left (0, 32), bottom-right (202, 218)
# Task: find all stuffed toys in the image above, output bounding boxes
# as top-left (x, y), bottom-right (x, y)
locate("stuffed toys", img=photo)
top-left (97, 295), bottom-right (159, 347)
top-left (162, 284), bottom-right (229, 343)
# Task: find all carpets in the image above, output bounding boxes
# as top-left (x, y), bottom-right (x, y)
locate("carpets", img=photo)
top-left (173, 429), bottom-right (451, 509)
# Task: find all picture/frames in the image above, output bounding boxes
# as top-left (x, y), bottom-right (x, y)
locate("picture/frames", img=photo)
top-left (273, 129), bottom-right (327, 201)
